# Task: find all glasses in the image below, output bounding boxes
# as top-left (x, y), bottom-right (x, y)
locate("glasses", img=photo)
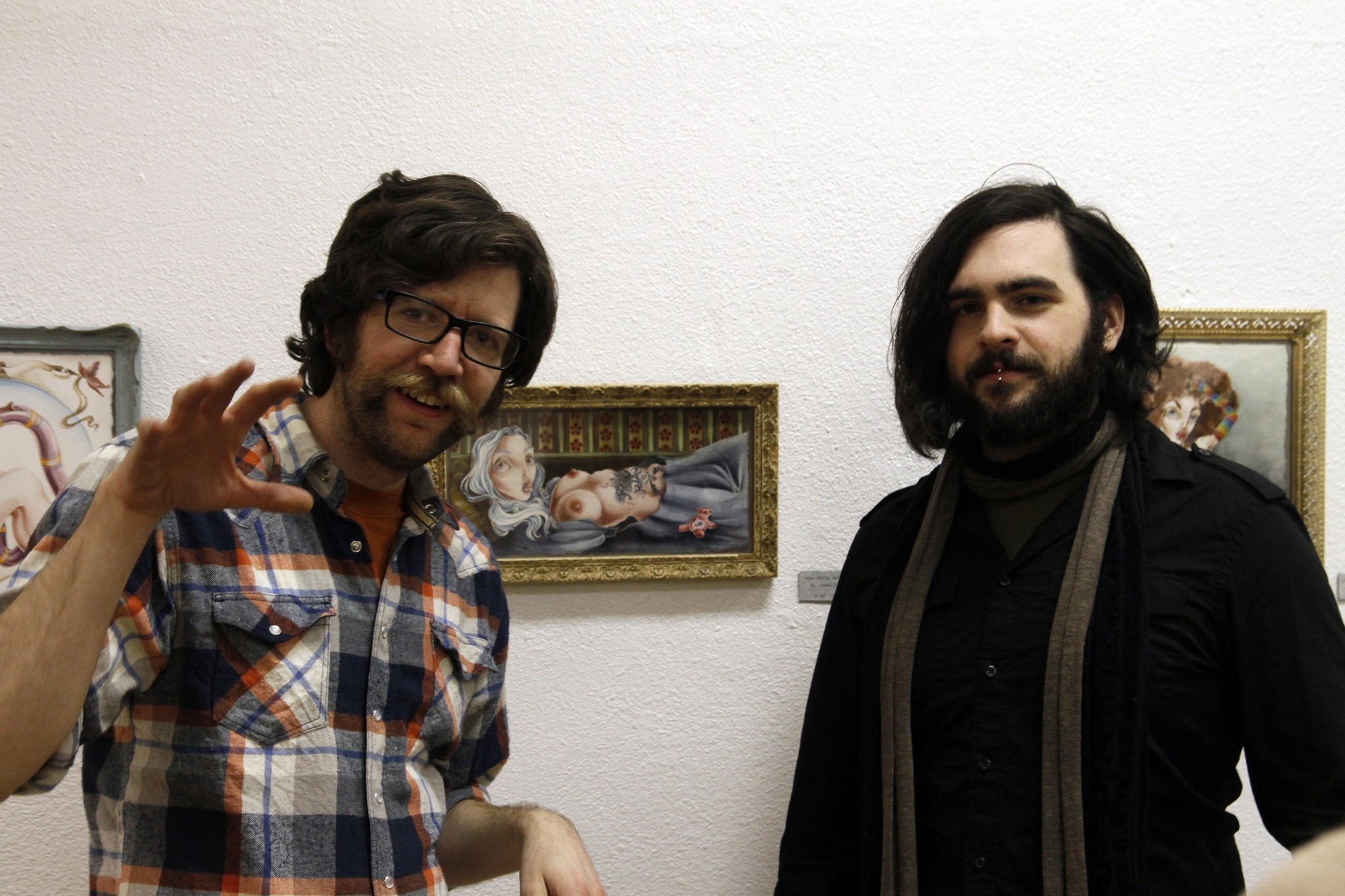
top-left (375, 288), bottom-right (526, 371)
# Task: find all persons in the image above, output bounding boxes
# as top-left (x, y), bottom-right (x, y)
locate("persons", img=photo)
top-left (0, 168), bottom-right (607, 896)
top-left (772, 183), bottom-right (1345, 896)
